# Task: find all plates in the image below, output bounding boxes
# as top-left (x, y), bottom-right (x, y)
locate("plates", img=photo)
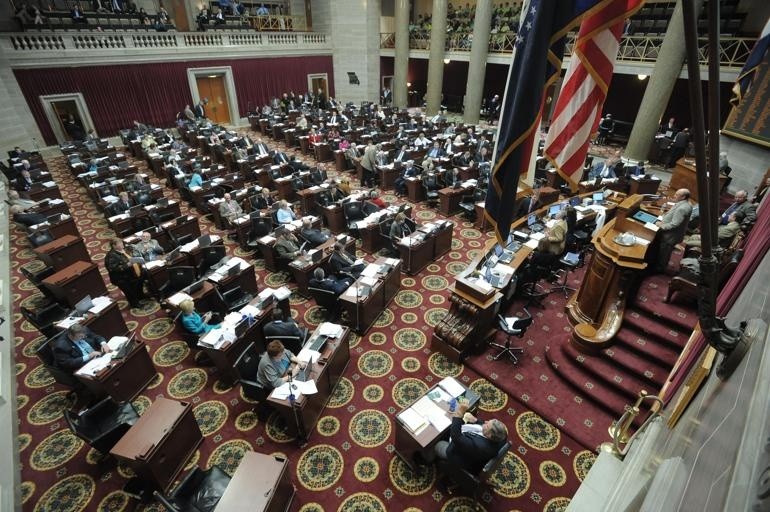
top-left (612, 236), bottom-right (636, 246)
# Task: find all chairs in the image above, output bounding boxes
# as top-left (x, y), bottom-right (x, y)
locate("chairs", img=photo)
top-left (622, 0), bottom-right (746, 66)
top-left (723, 167), bottom-right (732, 193)
top-left (0, 0), bottom-right (294, 31)
top-left (664, 201), bottom-right (754, 305)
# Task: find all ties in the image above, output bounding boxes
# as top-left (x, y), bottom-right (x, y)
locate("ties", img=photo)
top-left (79, 340), bottom-right (90, 353)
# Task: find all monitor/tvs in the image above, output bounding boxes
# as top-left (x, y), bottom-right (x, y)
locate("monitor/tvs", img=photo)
top-left (628, 208), bottom-right (658, 225)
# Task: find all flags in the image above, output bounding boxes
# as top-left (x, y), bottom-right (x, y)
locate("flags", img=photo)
top-left (727, 16), bottom-right (769, 109)
top-left (482, 2), bottom-right (612, 251)
top-left (541, 1), bottom-right (654, 195)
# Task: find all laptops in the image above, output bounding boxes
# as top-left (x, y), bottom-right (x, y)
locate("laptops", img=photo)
top-left (7, 101), bottom-right (604, 263)
top-left (485, 266), bottom-right (500, 287)
top-left (228, 262), bottom-right (241, 276)
top-left (295, 356), bottom-right (313, 381)
top-left (183, 279), bottom-right (204, 295)
top-left (310, 338), bottom-right (329, 352)
top-left (234, 317), bottom-right (250, 337)
top-left (257, 294), bottom-right (273, 309)
top-left (111, 332), bottom-right (136, 360)
top-left (69, 294), bottom-right (94, 317)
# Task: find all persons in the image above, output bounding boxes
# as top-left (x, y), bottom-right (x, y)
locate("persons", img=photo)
top-left (256, 339), bottom-right (305, 391)
top-left (623, 18), bottom-right (636, 37)
top-left (408, 1), bottom-right (522, 56)
top-left (2, 1), bottom-right (291, 29)
top-left (307, 266), bottom-right (353, 299)
top-left (0, 86), bottom-right (766, 283)
top-left (261, 307), bottom-right (305, 352)
top-left (50, 321), bottom-right (111, 373)
top-left (104, 236), bottom-right (150, 308)
top-left (433, 398), bottom-right (510, 473)
top-left (179, 300), bottom-right (225, 335)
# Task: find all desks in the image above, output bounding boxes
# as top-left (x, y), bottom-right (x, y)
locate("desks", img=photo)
top-left (668, 157), bottom-right (726, 206)
top-left (10, 90), bottom-right (662, 511)
